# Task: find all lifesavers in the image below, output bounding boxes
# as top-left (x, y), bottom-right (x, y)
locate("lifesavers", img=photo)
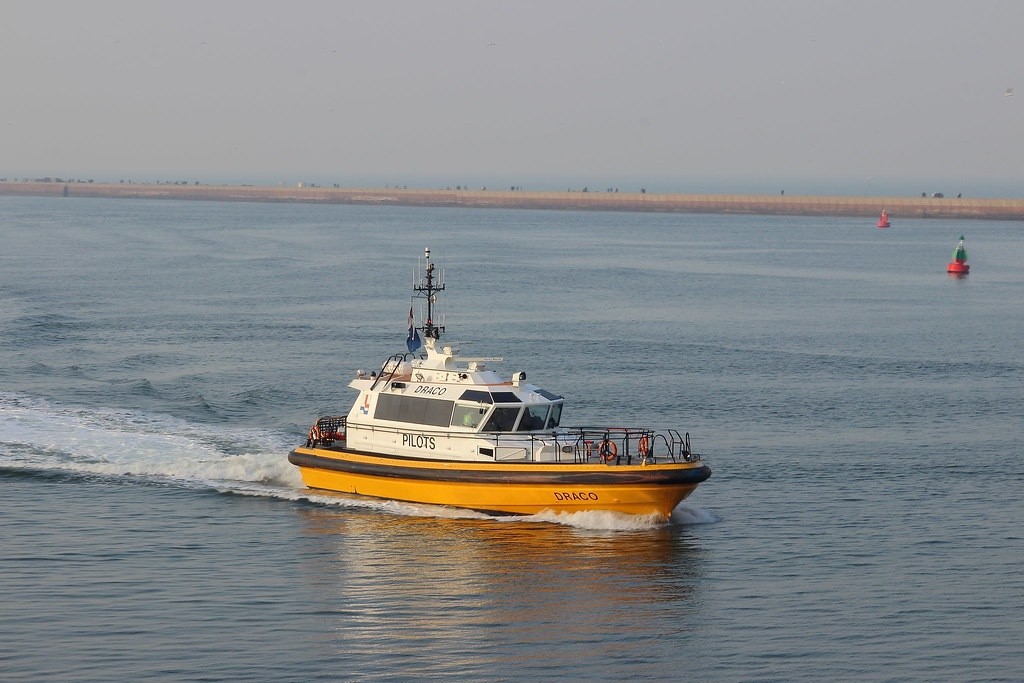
top-left (308, 425), bottom-right (321, 443)
top-left (638, 438), bottom-right (647, 458)
top-left (598, 440), bottom-right (617, 461)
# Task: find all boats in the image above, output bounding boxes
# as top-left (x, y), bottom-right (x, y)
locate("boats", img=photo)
top-left (287, 245), bottom-right (712, 526)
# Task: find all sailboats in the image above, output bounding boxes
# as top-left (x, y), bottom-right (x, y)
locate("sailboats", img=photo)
top-left (876, 207), bottom-right (891, 227)
top-left (946, 235), bottom-right (971, 274)
